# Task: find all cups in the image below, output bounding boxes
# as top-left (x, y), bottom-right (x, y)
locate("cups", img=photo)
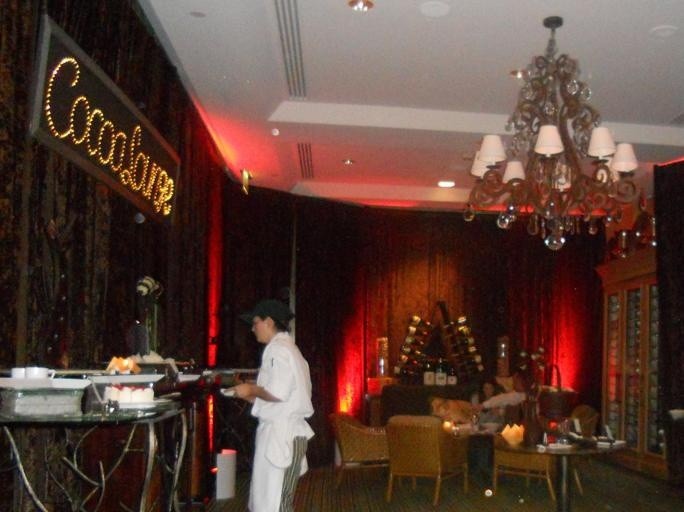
top-left (11, 367), bottom-right (25, 378)
top-left (25, 366), bottom-right (55, 379)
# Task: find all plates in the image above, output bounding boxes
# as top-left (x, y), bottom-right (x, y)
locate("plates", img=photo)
top-left (547, 445), bottom-right (572, 450)
top-left (597, 440), bottom-right (628, 447)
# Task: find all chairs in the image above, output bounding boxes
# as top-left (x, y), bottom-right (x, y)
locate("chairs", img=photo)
top-left (328, 394), bottom-right (627, 512)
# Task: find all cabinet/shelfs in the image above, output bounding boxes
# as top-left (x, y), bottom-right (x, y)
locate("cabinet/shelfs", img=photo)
top-left (595, 248), bottom-right (684, 482)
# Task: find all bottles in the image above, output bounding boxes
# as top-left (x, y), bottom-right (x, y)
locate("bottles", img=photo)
top-left (443, 316), bottom-right (485, 379)
top-left (393, 315), bottom-right (434, 378)
top-left (424, 359), bottom-right (458, 387)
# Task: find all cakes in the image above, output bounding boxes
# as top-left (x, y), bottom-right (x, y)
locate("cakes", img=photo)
top-left (103, 384), bottom-right (156, 403)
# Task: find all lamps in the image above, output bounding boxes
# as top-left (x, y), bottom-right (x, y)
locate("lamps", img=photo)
top-left (462, 17), bottom-right (638, 250)
top-left (135, 274), bottom-right (161, 356)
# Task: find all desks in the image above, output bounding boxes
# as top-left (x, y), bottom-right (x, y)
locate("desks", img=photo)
top-left (0, 398), bottom-right (190, 511)
top-left (380, 381), bottom-right (496, 432)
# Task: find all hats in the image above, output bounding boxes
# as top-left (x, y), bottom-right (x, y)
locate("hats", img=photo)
top-left (240, 299), bottom-right (293, 331)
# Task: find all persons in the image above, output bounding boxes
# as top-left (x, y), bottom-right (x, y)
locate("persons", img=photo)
top-left (463, 371), bottom-right (513, 497)
top-left (215, 294), bottom-right (316, 510)
